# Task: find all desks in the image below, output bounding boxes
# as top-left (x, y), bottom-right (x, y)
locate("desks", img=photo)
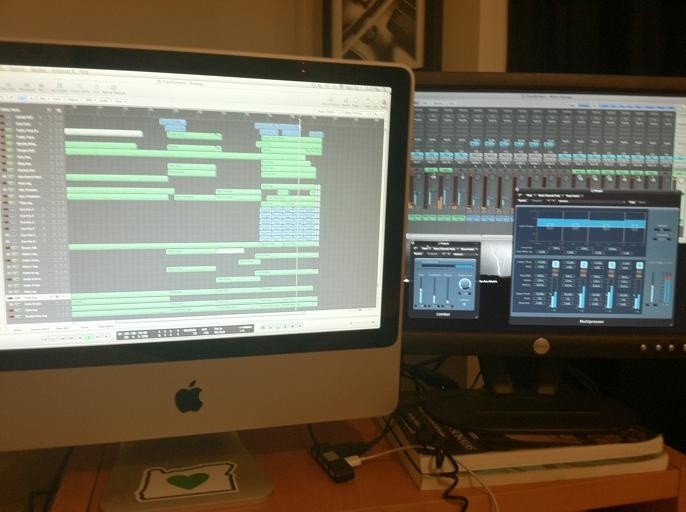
top-left (2, 417), bottom-right (683, 510)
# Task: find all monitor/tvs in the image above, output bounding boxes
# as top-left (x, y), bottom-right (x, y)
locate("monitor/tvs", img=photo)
top-left (0, 38), bottom-right (415, 509)
top-left (411, 68), bottom-right (686, 435)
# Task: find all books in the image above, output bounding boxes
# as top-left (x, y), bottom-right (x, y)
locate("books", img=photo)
top-left (382, 386), bottom-right (666, 473)
top-left (385, 430), bottom-right (670, 491)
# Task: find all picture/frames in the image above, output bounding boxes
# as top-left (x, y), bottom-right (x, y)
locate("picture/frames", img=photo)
top-left (323, 0), bottom-right (443, 73)
